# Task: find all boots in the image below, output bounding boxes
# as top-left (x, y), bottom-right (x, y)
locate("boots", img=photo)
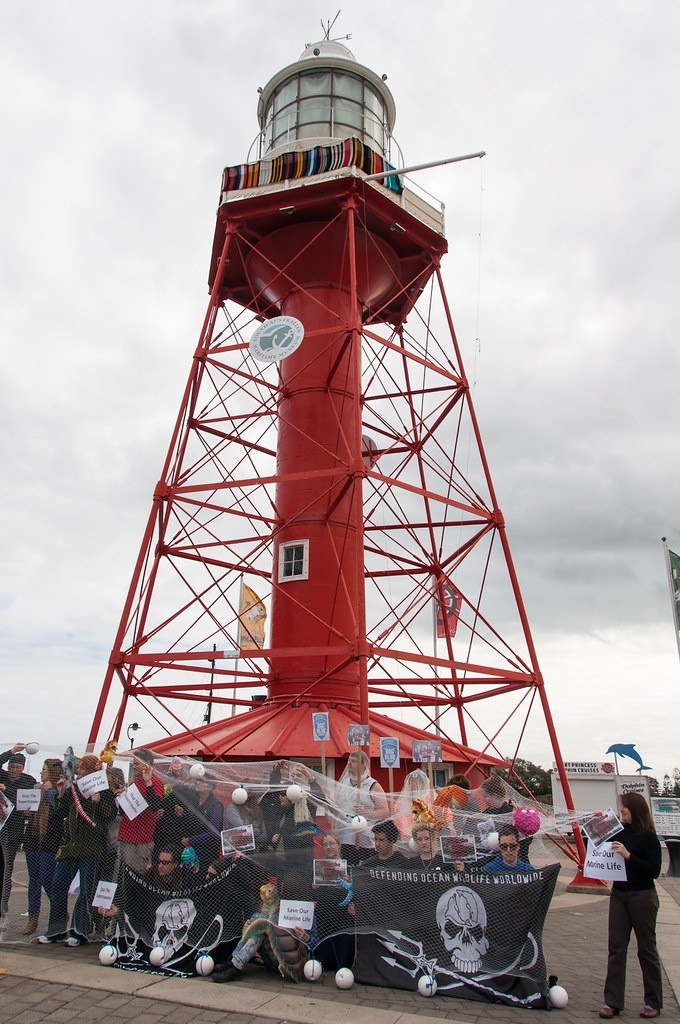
top-left (23, 911), bottom-right (40, 935)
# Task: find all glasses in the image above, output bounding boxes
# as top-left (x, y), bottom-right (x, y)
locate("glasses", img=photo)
top-left (499, 842), bottom-right (519, 851)
top-left (195, 779), bottom-right (209, 784)
top-left (158, 859), bottom-right (173, 866)
top-left (277, 793), bottom-right (286, 797)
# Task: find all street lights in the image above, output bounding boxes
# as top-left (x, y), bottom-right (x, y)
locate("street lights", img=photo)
top-left (127, 723), bottom-right (140, 749)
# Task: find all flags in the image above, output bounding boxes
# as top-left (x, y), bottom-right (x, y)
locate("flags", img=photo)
top-left (108, 860), bottom-right (243, 980)
top-left (350, 862), bottom-right (562, 1012)
top-left (240, 582), bottom-right (267, 650)
top-left (667, 547), bottom-right (680, 631)
top-left (435, 576), bottom-right (462, 638)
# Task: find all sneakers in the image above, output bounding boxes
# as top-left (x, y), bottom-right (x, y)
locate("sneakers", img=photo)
top-left (37, 933), bottom-right (68, 944)
top-left (64, 937), bottom-right (86, 946)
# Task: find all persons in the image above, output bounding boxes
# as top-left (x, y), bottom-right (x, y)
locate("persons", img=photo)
top-left (0, 743), bottom-right (37, 930)
top-left (37, 755), bottom-right (119, 946)
top-left (90, 750), bottom-right (536, 984)
top-left (591, 793), bottom-right (664, 1018)
top-left (22, 759), bottom-right (69, 936)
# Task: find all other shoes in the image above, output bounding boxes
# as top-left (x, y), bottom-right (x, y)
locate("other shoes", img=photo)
top-left (599, 1004), bottom-right (621, 1019)
top-left (640, 1007), bottom-right (661, 1018)
top-left (211, 960), bottom-right (242, 983)
